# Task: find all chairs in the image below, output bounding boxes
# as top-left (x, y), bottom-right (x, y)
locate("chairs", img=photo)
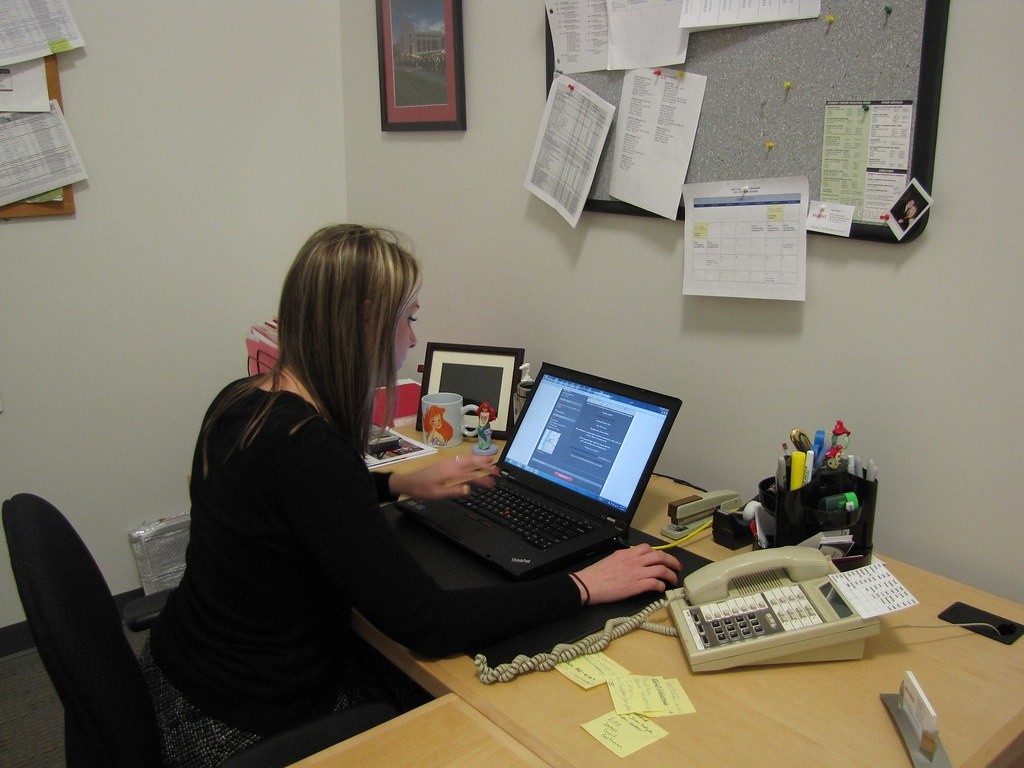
top-left (2, 493), bottom-right (398, 768)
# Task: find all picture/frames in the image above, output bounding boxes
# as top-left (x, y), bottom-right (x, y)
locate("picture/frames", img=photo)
top-left (375, 0), bottom-right (466, 132)
top-left (415, 342), bottom-right (525, 441)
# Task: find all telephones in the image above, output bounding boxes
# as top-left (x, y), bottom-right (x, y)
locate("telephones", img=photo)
top-left (665, 546), bottom-right (881, 672)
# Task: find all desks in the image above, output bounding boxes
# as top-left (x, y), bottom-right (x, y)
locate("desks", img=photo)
top-left (346, 421), bottom-right (1024, 768)
top-left (286, 690), bottom-right (551, 768)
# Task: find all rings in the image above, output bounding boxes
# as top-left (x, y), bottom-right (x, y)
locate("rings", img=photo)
top-left (455, 455), bottom-right (462, 462)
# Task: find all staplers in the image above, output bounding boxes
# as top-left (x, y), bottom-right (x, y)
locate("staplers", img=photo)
top-left (661, 488), bottom-right (743, 541)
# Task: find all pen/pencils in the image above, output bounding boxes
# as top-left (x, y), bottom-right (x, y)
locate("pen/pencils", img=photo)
top-left (847, 454), bottom-right (879, 484)
top-left (777, 442), bottom-right (815, 491)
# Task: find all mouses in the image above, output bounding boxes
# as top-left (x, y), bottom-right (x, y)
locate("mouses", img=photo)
top-left (655, 568), bottom-right (681, 591)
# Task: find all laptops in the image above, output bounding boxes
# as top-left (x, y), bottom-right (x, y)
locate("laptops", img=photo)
top-left (397, 362), bottom-right (683, 581)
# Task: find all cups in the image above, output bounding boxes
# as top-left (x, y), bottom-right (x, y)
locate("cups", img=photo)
top-left (518, 380), bottom-right (535, 413)
top-left (421, 392), bottom-right (480, 448)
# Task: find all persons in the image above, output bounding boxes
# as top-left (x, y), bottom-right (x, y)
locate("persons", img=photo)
top-left (476, 402), bottom-right (497, 450)
top-left (138, 224), bottom-right (683, 767)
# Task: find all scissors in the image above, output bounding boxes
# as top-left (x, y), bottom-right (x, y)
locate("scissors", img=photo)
top-left (811, 431), bottom-right (826, 477)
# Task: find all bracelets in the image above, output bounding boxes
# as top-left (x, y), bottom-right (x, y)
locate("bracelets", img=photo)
top-left (568, 571), bottom-right (590, 609)
